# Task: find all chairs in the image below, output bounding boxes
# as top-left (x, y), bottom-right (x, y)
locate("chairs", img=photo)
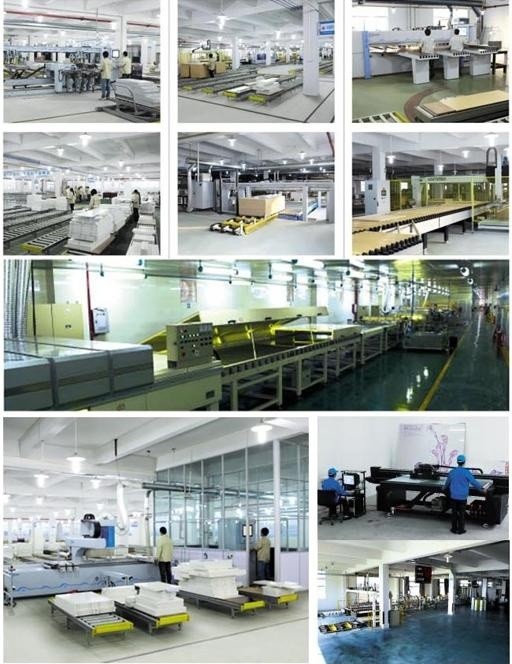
top-left (317, 489), bottom-right (344, 526)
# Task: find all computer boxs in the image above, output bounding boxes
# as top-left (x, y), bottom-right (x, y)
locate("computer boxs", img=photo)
top-left (349, 498), bottom-right (363, 517)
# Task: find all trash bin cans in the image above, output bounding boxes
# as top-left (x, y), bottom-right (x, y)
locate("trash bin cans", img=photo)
top-left (390, 610), bottom-right (401, 627)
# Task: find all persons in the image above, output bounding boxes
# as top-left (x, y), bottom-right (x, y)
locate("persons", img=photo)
top-left (115, 51), bottom-right (132, 78)
top-left (63, 183), bottom-right (101, 213)
top-left (420, 28), bottom-right (435, 78)
top-left (440, 454), bottom-right (487, 535)
top-left (96, 51), bottom-right (114, 101)
top-left (130, 189), bottom-right (141, 223)
top-left (205, 53), bottom-right (216, 77)
top-left (446, 28), bottom-right (464, 77)
top-left (155, 526), bottom-right (173, 585)
top-left (253, 527), bottom-right (272, 581)
top-left (320, 467), bottom-right (353, 521)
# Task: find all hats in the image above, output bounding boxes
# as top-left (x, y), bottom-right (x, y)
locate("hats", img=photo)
top-left (328, 468), bottom-right (338, 475)
top-left (457, 455), bottom-right (465, 462)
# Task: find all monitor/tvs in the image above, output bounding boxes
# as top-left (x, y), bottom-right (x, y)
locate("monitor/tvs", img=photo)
top-left (242, 525), bottom-right (253, 537)
top-left (458, 28), bottom-right (467, 36)
top-left (342, 473), bottom-right (360, 490)
top-left (112, 50), bottom-right (120, 58)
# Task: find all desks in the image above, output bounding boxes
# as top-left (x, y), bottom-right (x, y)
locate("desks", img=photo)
top-left (337, 490), bottom-right (366, 518)
